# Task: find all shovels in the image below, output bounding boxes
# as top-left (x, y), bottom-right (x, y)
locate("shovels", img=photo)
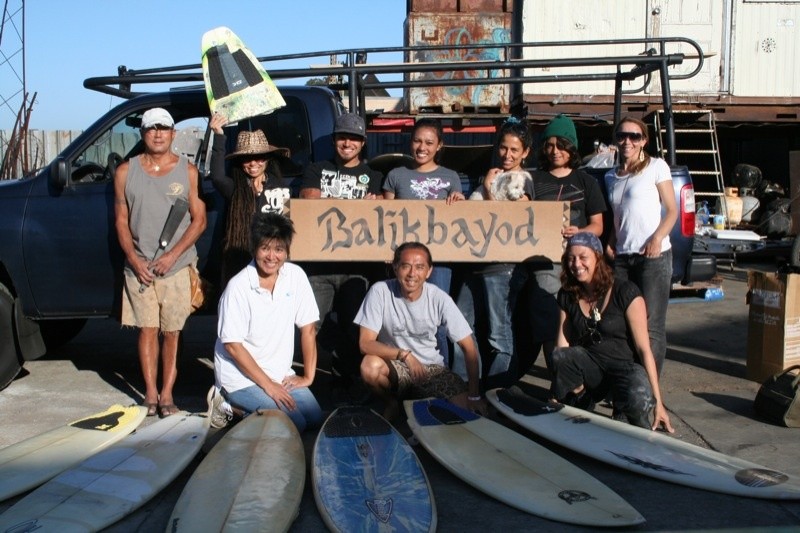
top-left (138, 198), bottom-right (190, 293)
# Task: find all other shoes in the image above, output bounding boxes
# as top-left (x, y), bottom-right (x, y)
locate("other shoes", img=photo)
top-left (564, 389), bottom-right (594, 413)
top-left (612, 399), bottom-right (629, 424)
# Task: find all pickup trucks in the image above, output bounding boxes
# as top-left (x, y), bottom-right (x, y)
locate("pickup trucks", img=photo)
top-left (1, 38), bottom-right (713, 395)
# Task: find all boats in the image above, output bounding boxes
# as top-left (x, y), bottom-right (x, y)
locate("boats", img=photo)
top-left (692, 224), bottom-right (768, 256)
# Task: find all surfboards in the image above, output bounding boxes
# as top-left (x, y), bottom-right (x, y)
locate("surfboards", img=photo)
top-left (0, 410), bottom-right (213, 533)
top-left (486, 386), bottom-right (796, 499)
top-left (165, 408), bottom-right (306, 533)
top-left (402, 395), bottom-right (649, 527)
top-left (0, 403), bottom-right (149, 500)
top-left (311, 404), bottom-right (439, 533)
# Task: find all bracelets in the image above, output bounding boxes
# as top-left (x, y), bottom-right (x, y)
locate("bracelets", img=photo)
top-left (466, 396), bottom-right (481, 401)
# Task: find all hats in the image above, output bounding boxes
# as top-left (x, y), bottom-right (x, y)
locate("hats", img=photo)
top-left (141, 108), bottom-right (174, 129)
top-left (334, 114), bottom-right (366, 139)
top-left (538, 114), bottom-right (577, 149)
top-left (224, 130), bottom-right (290, 160)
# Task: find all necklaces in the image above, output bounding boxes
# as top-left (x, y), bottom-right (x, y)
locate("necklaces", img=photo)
top-left (153, 165), bottom-right (161, 171)
top-left (587, 294), bottom-right (602, 321)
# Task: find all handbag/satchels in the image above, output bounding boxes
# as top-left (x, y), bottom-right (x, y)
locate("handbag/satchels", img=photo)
top-left (753, 364), bottom-right (800, 428)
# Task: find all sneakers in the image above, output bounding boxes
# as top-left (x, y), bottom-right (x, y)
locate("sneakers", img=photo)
top-left (207, 386), bottom-right (229, 428)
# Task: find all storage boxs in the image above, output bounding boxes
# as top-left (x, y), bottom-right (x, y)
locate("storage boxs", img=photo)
top-left (746, 269), bottom-right (800, 383)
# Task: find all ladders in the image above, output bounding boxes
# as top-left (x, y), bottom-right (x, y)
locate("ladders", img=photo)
top-left (654, 109), bottom-right (731, 230)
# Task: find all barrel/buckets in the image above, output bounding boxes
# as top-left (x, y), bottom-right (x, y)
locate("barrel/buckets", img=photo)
top-left (660, 166), bottom-right (695, 283)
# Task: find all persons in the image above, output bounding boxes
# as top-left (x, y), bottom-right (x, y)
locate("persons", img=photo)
top-left (207, 215), bottom-right (322, 434)
top-left (473, 118), bottom-right (534, 377)
top-left (384, 118), bottom-right (466, 366)
top-left (298, 113), bottom-right (384, 370)
top-left (208, 111), bottom-right (294, 310)
top-left (606, 117), bottom-right (679, 383)
top-left (535, 112), bottom-right (607, 371)
top-left (351, 241), bottom-right (488, 419)
top-left (114, 107), bottom-right (206, 418)
top-left (550, 231), bottom-right (675, 434)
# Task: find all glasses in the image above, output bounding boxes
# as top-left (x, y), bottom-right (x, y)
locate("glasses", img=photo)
top-left (586, 318), bottom-right (602, 345)
top-left (616, 131), bottom-right (644, 143)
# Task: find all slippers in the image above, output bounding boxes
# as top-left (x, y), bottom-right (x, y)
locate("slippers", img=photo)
top-left (142, 402), bottom-right (157, 417)
top-left (158, 402), bottom-right (177, 418)
